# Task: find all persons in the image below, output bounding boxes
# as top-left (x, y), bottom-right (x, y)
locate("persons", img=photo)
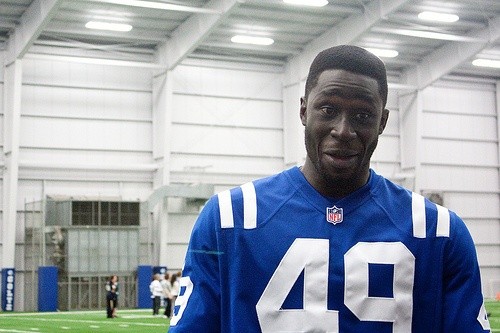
top-left (162, 273), bottom-right (171, 318)
top-left (107, 285), bottom-right (118, 318)
top-left (150, 273), bottom-right (162, 315)
top-left (169, 44), bottom-right (492, 333)
top-left (106, 275), bottom-right (118, 318)
top-left (177, 272), bottom-right (182, 284)
top-left (171, 274), bottom-right (179, 301)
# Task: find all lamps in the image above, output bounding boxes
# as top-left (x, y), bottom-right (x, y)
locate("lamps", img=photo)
top-left (85, 21), bottom-right (133, 32)
top-left (364, 47), bottom-right (399, 57)
top-left (282, 0), bottom-right (329, 6)
top-left (472, 57), bottom-right (500, 68)
top-left (231, 34), bottom-right (275, 46)
top-left (418, 9), bottom-right (459, 22)
